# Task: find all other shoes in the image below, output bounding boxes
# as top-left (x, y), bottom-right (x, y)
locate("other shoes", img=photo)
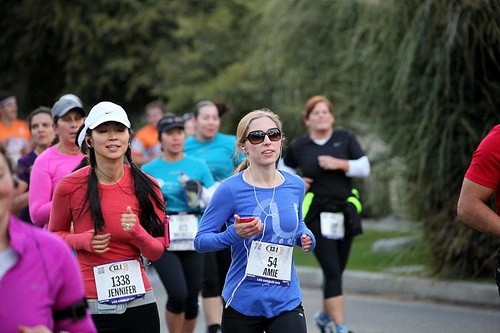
top-left (334, 323), bottom-right (352, 333)
top-left (316, 311), bottom-right (334, 333)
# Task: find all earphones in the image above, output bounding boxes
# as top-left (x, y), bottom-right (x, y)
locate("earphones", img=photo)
top-left (87, 140), bottom-right (91, 145)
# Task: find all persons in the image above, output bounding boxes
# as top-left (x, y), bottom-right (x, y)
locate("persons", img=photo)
top-left (457, 124), bottom-right (500, 238)
top-left (48, 102), bottom-right (170, 333)
top-left (0, 144), bottom-right (97, 333)
top-left (282, 96), bottom-right (369, 333)
top-left (194, 110), bottom-right (315, 333)
top-left (130, 103), bottom-right (166, 167)
top-left (184, 100), bottom-right (248, 333)
top-left (13, 107), bottom-right (56, 224)
top-left (141, 114), bottom-right (214, 333)
top-left (0, 95), bottom-right (34, 172)
top-left (28, 93), bottom-right (89, 231)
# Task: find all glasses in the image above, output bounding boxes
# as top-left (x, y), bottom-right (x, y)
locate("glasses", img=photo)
top-left (159, 116), bottom-right (185, 128)
top-left (242, 127), bottom-right (281, 144)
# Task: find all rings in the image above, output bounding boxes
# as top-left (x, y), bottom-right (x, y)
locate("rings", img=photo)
top-left (126, 224), bottom-right (129, 229)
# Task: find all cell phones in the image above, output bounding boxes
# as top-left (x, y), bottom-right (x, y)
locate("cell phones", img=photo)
top-left (237, 217), bottom-right (256, 223)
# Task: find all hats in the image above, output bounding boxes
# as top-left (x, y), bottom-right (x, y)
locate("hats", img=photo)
top-left (51, 93), bottom-right (86, 120)
top-left (156, 116), bottom-right (183, 131)
top-left (85, 101), bottom-right (131, 132)
